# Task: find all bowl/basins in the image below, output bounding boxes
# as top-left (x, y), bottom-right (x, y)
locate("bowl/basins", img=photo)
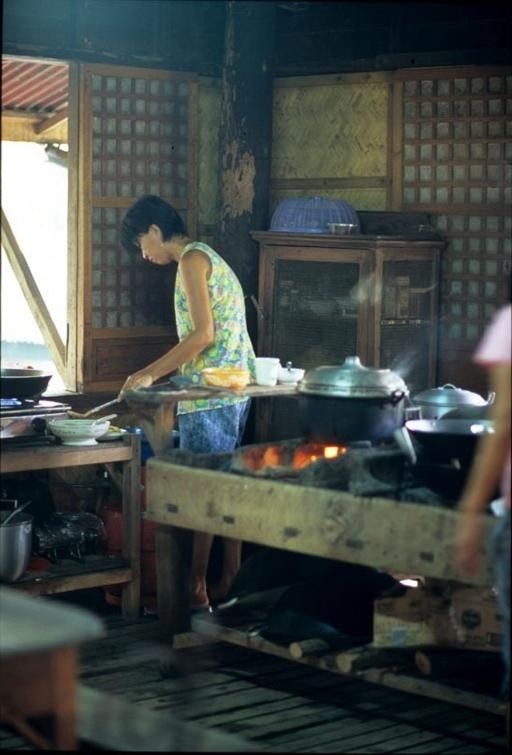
top-left (48, 419), bottom-right (111, 447)
top-left (328, 222), bottom-right (359, 236)
top-left (277, 367), bottom-right (305, 388)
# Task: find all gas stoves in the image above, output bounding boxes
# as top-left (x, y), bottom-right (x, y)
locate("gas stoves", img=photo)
top-left (0, 397), bottom-right (72, 439)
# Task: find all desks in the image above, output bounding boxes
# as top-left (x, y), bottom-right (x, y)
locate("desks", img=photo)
top-left (1, 581), bottom-right (105, 755)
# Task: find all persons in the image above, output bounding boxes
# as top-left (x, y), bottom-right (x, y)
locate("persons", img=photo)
top-left (118, 194), bottom-right (262, 609)
top-left (457, 305), bottom-right (509, 684)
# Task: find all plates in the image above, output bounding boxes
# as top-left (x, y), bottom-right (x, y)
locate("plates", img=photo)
top-left (96, 428), bottom-right (128, 442)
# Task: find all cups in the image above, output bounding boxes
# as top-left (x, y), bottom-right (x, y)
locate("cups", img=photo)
top-left (255, 357), bottom-right (280, 386)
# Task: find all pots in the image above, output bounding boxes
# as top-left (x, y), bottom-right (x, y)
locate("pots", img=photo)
top-left (412, 382), bottom-right (496, 420)
top-left (294, 354), bottom-right (410, 448)
top-left (0, 498), bottom-right (35, 584)
top-left (0, 368), bottom-right (53, 401)
top-left (405, 418), bottom-right (502, 501)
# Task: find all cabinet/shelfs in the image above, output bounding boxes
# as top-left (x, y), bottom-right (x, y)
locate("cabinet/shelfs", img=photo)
top-left (1, 433), bottom-right (140, 619)
top-left (250, 229), bottom-right (447, 444)
